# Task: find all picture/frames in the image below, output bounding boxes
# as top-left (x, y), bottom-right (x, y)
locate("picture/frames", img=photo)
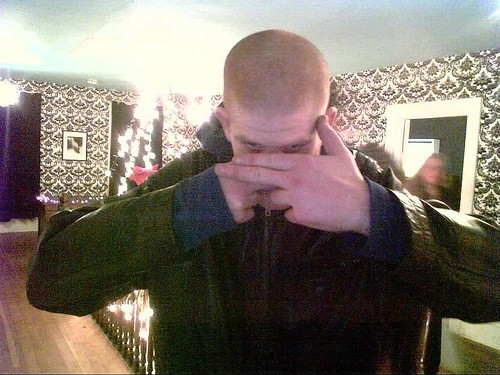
top-left (62, 130), bottom-right (87, 161)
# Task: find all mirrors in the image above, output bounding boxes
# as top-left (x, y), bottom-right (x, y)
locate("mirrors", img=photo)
top-left (384, 96), bottom-right (482, 213)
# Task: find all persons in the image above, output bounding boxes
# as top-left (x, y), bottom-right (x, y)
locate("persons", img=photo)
top-left (26, 30), bottom-right (500, 375)
top-left (406, 151), bottom-right (462, 215)
